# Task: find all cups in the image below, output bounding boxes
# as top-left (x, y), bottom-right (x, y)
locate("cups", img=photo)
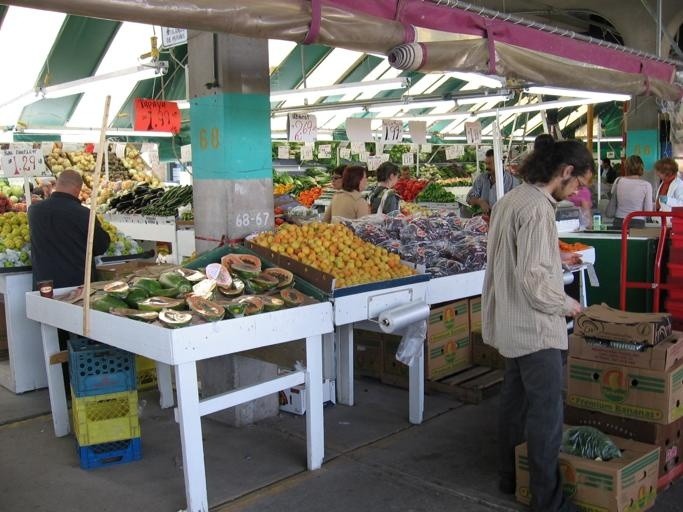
top-left (600, 225), bottom-right (608, 231)
top-left (37, 279), bottom-right (54, 300)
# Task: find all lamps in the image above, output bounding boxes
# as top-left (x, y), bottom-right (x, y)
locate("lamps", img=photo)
top-left (0, 37), bottom-right (169, 121)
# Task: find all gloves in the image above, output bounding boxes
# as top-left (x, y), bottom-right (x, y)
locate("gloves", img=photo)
top-left (659, 195), bottom-right (667, 202)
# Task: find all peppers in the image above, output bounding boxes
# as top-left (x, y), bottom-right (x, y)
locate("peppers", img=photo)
top-left (396, 178), bottom-right (455, 204)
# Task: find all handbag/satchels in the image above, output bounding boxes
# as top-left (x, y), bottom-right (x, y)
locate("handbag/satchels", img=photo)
top-left (606, 193), bottom-right (617, 218)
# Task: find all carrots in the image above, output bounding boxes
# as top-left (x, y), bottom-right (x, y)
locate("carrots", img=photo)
top-left (300, 187), bottom-right (321, 207)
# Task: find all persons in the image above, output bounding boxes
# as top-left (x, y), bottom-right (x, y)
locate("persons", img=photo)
top-left (479, 134), bottom-right (594, 511)
top-left (25, 169), bottom-right (110, 400)
top-left (609, 156), bottom-right (653, 230)
top-left (316, 166), bottom-right (371, 223)
top-left (329, 164), bottom-right (348, 191)
top-left (600, 158), bottom-right (615, 184)
top-left (652, 158), bottom-right (683, 226)
top-left (367, 160), bottom-right (399, 216)
top-left (465, 148), bottom-right (521, 216)
top-left (566, 187), bottom-right (593, 230)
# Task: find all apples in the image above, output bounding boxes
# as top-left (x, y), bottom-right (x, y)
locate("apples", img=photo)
top-left (0, 192), bottom-right (42, 268)
top-left (97, 214), bottom-right (143, 257)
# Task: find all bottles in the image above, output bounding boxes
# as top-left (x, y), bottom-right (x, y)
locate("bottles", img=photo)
top-left (593, 212), bottom-right (601, 231)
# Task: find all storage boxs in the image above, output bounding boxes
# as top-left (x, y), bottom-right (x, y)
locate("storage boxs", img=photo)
top-left (354, 296), bottom-right (501, 382)
top-left (515, 303), bottom-right (683, 512)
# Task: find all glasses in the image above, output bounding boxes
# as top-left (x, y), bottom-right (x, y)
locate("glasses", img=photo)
top-left (331, 176), bottom-right (342, 180)
top-left (576, 176), bottom-right (586, 189)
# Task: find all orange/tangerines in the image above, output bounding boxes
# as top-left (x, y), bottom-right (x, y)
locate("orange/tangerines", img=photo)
top-left (249, 222), bottom-right (417, 289)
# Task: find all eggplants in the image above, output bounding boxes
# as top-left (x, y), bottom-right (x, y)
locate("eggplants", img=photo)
top-left (110, 182), bottom-right (165, 214)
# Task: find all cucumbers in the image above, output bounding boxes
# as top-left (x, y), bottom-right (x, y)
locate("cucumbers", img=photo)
top-left (144, 185), bottom-right (192, 216)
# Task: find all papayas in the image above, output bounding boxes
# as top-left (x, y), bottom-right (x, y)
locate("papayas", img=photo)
top-left (89, 253), bottom-right (304, 329)
